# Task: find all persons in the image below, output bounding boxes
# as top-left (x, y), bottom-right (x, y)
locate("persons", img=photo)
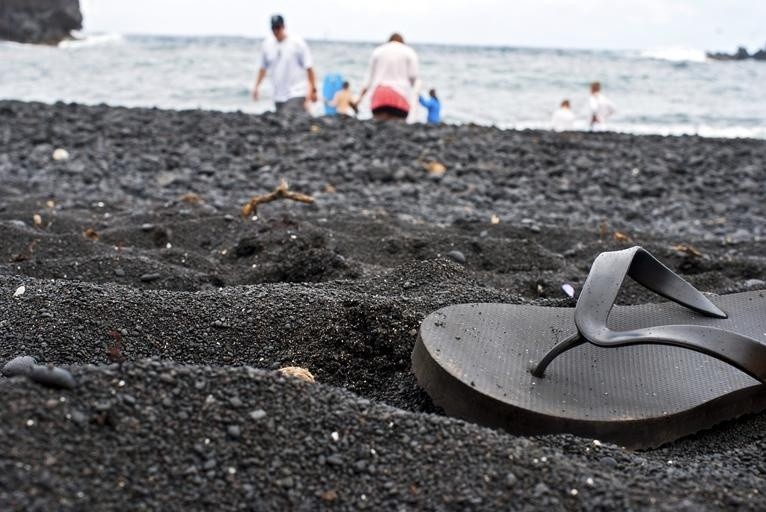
top-left (322, 81), bottom-right (354, 116)
top-left (416, 88), bottom-right (441, 124)
top-left (584, 80), bottom-right (615, 132)
top-left (551, 99), bottom-right (573, 130)
top-left (354, 32), bottom-right (420, 122)
top-left (251, 12), bottom-right (317, 115)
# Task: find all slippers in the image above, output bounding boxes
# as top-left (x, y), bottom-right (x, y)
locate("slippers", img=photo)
top-left (409, 246), bottom-right (761, 452)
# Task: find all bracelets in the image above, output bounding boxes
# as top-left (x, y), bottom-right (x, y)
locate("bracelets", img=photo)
top-left (311, 88), bottom-right (316, 92)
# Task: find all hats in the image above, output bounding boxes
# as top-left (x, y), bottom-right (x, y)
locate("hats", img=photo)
top-left (271, 15), bottom-right (284, 28)
